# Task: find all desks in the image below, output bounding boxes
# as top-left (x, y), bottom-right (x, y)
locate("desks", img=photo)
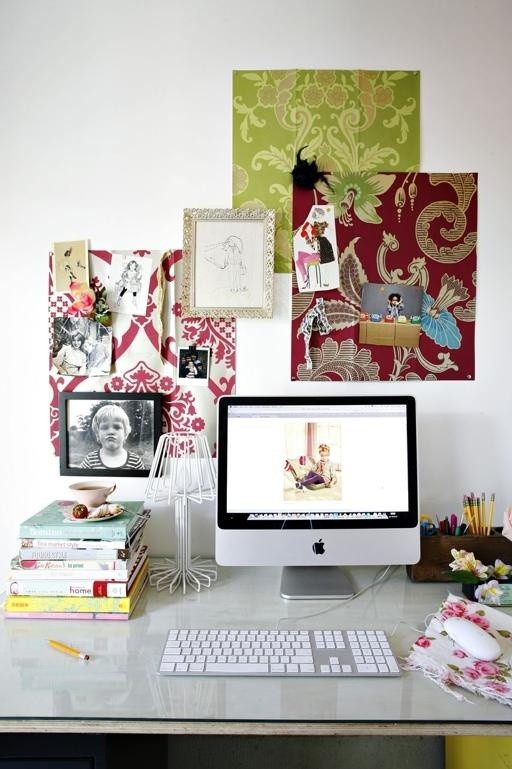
top-left (1, 556), bottom-right (512, 768)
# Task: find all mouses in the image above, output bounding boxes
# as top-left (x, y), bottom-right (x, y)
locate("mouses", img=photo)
top-left (444, 613), bottom-right (506, 662)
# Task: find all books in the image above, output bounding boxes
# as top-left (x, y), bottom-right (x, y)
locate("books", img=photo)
top-left (3, 499), bottom-right (152, 621)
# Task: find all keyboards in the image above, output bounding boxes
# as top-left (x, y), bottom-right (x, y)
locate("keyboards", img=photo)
top-left (158, 626), bottom-right (408, 676)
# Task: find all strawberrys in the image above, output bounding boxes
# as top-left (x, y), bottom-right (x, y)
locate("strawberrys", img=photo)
top-left (72, 504), bottom-right (88, 519)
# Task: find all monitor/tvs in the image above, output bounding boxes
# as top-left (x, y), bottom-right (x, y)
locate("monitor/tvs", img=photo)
top-left (214, 394), bottom-right (423, 599)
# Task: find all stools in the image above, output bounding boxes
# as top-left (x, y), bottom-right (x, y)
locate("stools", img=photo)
top-left (305, 261), bottom-right (322, 288)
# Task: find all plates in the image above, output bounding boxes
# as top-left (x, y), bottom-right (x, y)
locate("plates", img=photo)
top-left (60, 503), bottom-right (124, 524)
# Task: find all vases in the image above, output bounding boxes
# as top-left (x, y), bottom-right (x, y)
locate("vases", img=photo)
top-left (460, 581), bottom-right (486, 602)
top-left (461, 582), bottom-right (481, 602)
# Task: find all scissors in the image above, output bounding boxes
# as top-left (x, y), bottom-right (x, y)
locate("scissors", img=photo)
top-left (420, 518), bottom-right (437, 536)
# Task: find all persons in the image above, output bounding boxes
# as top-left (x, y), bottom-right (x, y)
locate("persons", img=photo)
top-left (53, 330), bottom-right (86, 375)
top-left (181, 355), bottom-right (202, 378)
top-left (79, 405), bottom-right (144, 469)
top-left (81, 341), bottom-right (110, 374)
top-left (295, 443), bottom-right (334, 488)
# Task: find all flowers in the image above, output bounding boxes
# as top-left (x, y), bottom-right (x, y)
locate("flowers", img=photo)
top-left (441, 546), bottom-right (511, 608)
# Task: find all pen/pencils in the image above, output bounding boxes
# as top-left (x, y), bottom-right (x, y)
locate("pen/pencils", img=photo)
top-left (435, 491), bottom-right (496, 537)
top-left (44, 637), bottom-right (90, 661)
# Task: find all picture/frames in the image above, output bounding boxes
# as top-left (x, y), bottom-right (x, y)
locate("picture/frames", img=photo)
top-left (181, 205), bottom-right (275, 322)
top-left (57, 392), bottom-right (165, 479)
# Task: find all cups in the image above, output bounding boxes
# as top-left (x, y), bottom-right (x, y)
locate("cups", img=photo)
top-left (68, 481), bottom-right (117, 508)
